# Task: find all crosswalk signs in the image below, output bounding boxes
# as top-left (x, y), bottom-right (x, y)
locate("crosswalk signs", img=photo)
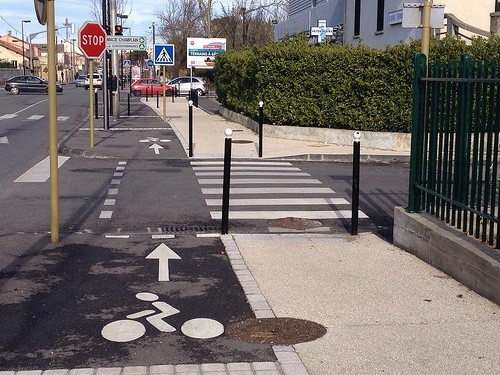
top-left (153, 44), bottom-right (176, 67)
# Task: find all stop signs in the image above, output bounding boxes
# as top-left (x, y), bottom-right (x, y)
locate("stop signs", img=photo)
top-left (78, 21), bottom-right (107, 60)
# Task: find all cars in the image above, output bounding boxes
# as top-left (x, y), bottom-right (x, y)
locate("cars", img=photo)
top-left (84, 74), bottom-right (103, 90)
top-left (5, 75), bottom-right (63, 95)
top-left (130, 78), bottom-right (177, 98)
top-left (165, 76), bottom-right (208, 96)
top-left (76, 75), bottom-right (88, 88)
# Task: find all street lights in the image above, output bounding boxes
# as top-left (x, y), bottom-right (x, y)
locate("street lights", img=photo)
top-left (21, 20), bottom-right (32, 73)
top-left (148, 21), bottom-right (156, 79)
top-left (118, 14), bottom-right (129, 89)
top-left (123, 27), bottom-right (132, 96)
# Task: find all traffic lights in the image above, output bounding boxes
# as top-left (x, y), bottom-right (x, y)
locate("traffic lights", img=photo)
top-left (115, 26), bottom-right (123, 36)
